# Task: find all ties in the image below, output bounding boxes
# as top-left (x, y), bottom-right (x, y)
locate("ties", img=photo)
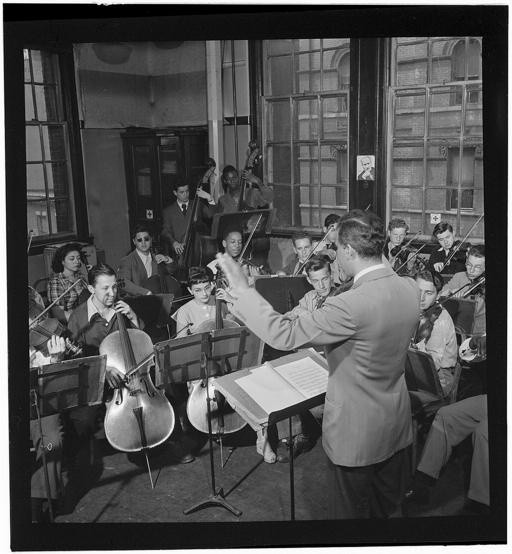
top-left (182, 204), bottom-right (187, 215)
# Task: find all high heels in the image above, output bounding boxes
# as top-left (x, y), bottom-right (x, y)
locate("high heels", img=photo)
top-left (256, 439), bottom-right (276, 464)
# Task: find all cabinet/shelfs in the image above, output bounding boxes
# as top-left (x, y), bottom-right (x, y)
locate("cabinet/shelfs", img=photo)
top-left (119, 128), bottom-right (208, 245)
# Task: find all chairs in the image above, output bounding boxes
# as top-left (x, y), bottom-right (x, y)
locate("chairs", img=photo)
top-left (441, 297), bottom-right (476, 403)
top-left (255, 274), bottom-right (314, 360)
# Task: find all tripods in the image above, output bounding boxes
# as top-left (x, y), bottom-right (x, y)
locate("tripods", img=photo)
top-left (154, 379), bottom-right (266, 518)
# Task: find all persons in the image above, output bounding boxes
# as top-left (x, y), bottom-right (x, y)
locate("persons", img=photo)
top-left (356, 154), bottom-right (376, 182)
top-left (30, 298), bottom-right (67, 524)
top-left (405, 268), bottom-right (457, 415)
top-left (215, 208), bottom-right (421, 520)
top-left (312, 213), bottom-right (347, 282)
top-left (161, 179), bottom-right (219, 255)
top-left (436, 242), bottom-right (487, 365)
top-left (275, 229), bottom-right (325, 277)
top-left (286, 256), bottom-right (343, 355)
top-left (404, 394), bottom-right (490, 518)
top-left (427, 221), bottom-right (471, 278)
top-left (48, 242), bottom-right (94, 325)
top-left (206, 225), bottom-right (266, 291)
top-left (115, 224), bottom-right (178, 296)
top-left (67, 262), bottom-right (194, 464)
top-left (383, 219), bottom-right (419, 280)
top-left (171, 266), bottom-right (277, 465)
top-left (212, 164), bottom-right (274, 212)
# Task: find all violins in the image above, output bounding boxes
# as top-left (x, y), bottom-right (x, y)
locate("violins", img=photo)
top-left (391, 242), bottom-right (445, 285)
top-left (28, 298), bottom-right (87, 357)
top-left (415, 301), bottom-right (441, 342)
top-left (450, 241), bottom-right (471, 259)
top-left (469, 278), bottom-right (485, 297)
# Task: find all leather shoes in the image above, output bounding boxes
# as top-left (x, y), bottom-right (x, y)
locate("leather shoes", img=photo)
top-left (277, 439), bottom-right (292, 462)
top-left (174, 447), bottom-right (194, 463)
top-left (294, 432), bottom-right (319, 452)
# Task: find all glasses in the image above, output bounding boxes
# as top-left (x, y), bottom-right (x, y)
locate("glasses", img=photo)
top-left (137, 237), bottom-right (150, 241)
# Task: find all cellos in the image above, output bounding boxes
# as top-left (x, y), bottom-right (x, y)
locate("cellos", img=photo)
top-left (183, 263), bottom-right (249, 437)
top-left (99, 266), bottom-right (175, 453)
top-left (143, 209), bottom-right (182, 294)
top-left (234, 141), bottom-right (266, 209)
top-left (172, 158), bottom-right (218, 280)
